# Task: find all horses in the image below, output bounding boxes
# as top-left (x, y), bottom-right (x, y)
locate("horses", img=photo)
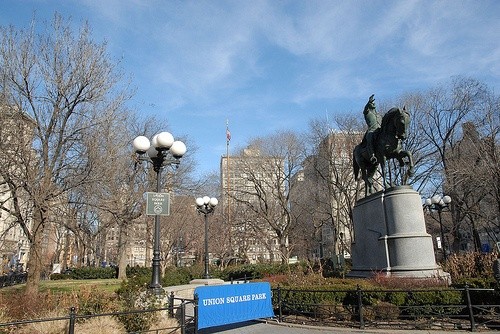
top-left (352, 106), bottom-right (416, 198)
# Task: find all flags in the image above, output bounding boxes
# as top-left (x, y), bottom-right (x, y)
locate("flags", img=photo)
top-left (226, 127), bottom-right (232, 145)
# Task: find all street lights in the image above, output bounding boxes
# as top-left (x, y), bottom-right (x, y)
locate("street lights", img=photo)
top-left (132, 131), bottom-right (186, 298)
top-left (196, 196), bottom-right (218, 279)
top-left (425, 194), bottom-right (451, 260)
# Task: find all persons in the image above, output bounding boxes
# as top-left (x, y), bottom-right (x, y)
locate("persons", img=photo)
top-left (362, 94), bottom-right (389, 166)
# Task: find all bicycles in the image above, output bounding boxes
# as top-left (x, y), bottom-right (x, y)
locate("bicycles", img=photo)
top-left (0, 270), bottom-right (28, 289)
